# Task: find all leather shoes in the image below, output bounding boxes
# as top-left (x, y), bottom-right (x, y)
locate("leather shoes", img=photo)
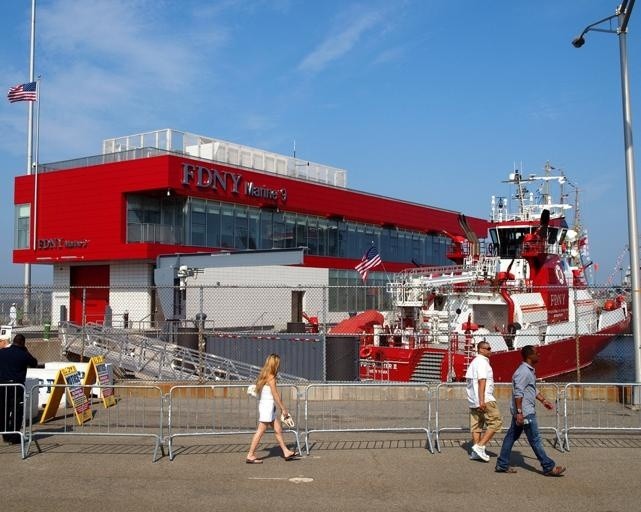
top-left (281, 413), bottom-right (294, 428)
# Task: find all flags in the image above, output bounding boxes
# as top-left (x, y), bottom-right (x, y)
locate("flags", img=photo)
top-left (5, 74), bottom-right (40, 250)
top-left (352, 241), bottom-right (395, 288)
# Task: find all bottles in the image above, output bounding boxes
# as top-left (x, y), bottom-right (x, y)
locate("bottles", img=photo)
top-left (516, 418), bottom-right (533, 425)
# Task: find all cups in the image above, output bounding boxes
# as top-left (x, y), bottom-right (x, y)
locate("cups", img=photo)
top-left (285, 416), bottom-right (294, 427)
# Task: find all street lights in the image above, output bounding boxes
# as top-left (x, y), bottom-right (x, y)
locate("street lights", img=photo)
top-left (572, 1), bottom-right (640, 407)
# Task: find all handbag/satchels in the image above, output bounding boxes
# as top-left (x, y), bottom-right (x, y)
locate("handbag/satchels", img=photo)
top-left (247, 385), bottom-right (259, 400)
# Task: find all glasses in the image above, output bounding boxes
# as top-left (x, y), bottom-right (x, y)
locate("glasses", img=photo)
top-left (482, 347), bottom-right (490, 351)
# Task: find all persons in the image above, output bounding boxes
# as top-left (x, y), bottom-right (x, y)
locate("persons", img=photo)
top-left (245, 353), bottom-right (300, 464)
top-left (464, 340), bottom-right (505, 464)
top-left (0, 334), bottom-right (38, 444)
top-left (496, 343), bottom-right (568, 478)
top-left (8, 302), bottom-right (21, 326)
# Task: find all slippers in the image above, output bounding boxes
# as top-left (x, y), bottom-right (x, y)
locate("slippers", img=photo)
top-left (246, 458), bottom-right (263, 464)
top-left (285, 451), bottom-right (299, 461)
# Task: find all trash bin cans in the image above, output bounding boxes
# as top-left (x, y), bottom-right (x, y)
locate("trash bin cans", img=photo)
top-left (25, 377), bottom-right (39, 420)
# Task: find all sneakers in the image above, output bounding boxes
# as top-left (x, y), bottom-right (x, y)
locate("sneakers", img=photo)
top-left (506, 469), bottom-right (516, 473)
top-left (544, 466), bottom-right (566, 476)
top-left (470, 444), bottom-right (489, 462)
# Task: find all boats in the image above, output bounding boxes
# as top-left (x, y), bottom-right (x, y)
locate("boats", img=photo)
top-left (302, 160), bottom-right (630, 384)
